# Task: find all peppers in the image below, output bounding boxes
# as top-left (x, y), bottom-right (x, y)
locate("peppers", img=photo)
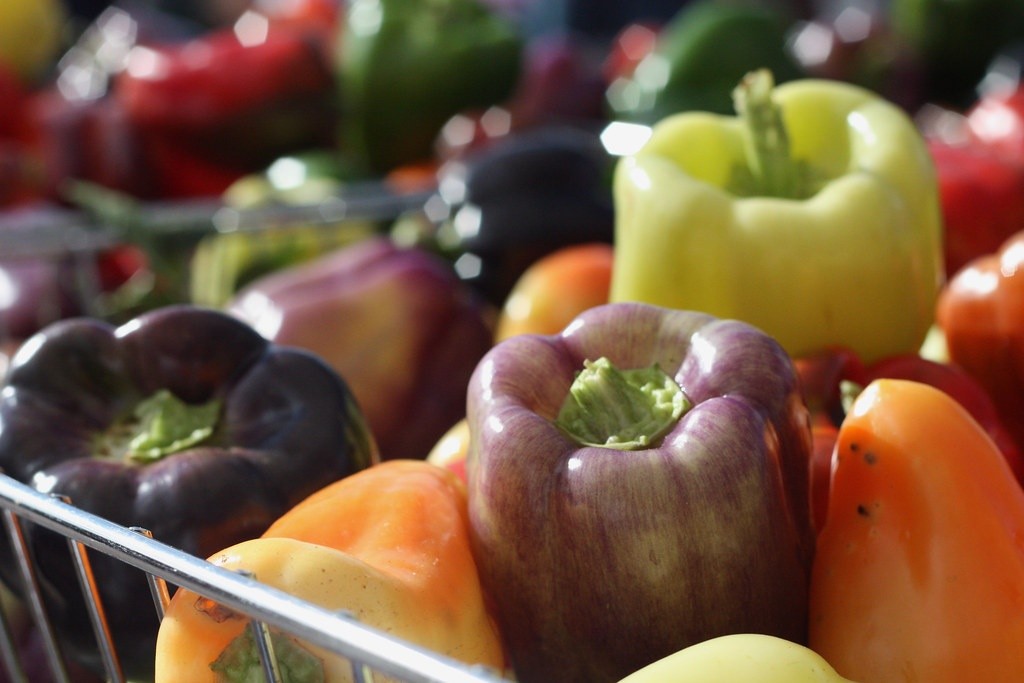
top-left (0, 0), bottom-right (1024, 683)
